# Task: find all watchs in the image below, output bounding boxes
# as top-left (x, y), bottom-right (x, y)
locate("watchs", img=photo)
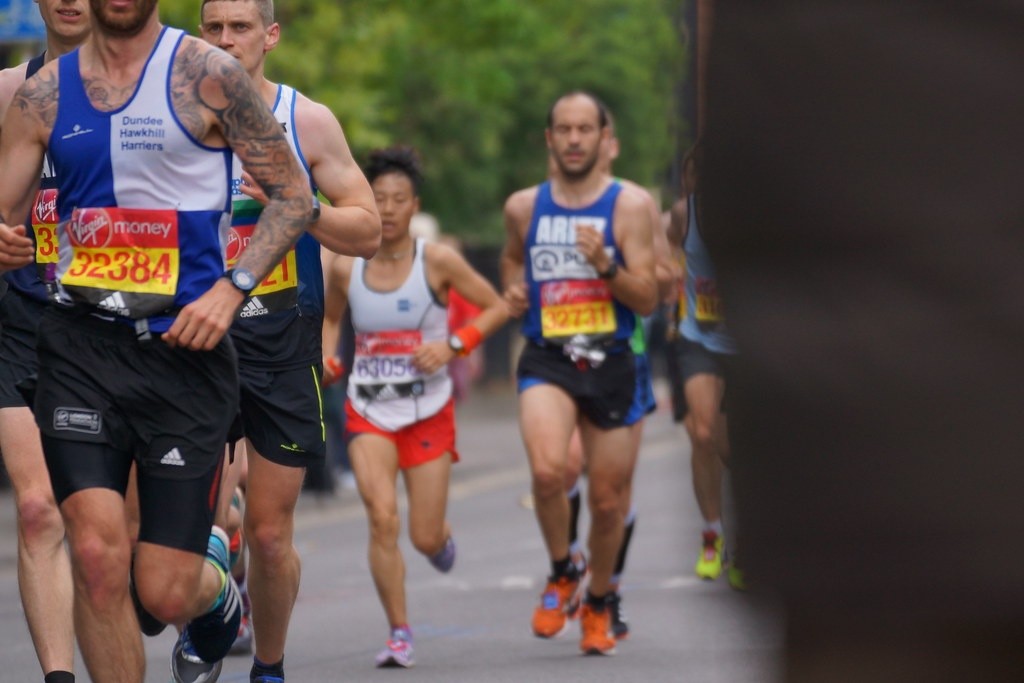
top-left (448, 334), bottom-right (464, 351)
top-left (223, 269), bottom-right (256, 302)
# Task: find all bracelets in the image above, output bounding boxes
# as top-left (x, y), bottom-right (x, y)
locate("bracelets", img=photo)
top-left (310, 195), bottom-right (321, 224)
top-left (599, 261), bottom-right (616, 278)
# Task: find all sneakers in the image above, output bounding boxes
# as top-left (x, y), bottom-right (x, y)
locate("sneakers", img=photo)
top-left (695, 530), bottom-right (724, 581)
top-left (532, 565), bottom-right (580, 639)
top-left (170, 628), bottom-right (223, 683)
top-left (224, 590), bottom-right (251, 655)
top-left (185, 527), bottom-right (242, 664)
top-left (376, 633), bottom-right (416, 668)
top-left (727, 544), bottom-right (746, 590)
top-left (432, 535), bottom-right (455, 573)
top-left (581, 597), bottom-right (617, 657)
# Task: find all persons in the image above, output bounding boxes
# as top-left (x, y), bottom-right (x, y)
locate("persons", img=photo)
top-left (308, 148), bottom-right (508, 668)
top-left (662, 153), bottom-right (747, 589)
top-left (0, 0), bottom-right (313, 683)
top-left (504, 92), bottom-right (673, 656)
top-left (198, 0), bottom-right (382, 683)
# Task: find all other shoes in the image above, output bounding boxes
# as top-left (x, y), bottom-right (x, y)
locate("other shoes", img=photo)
top-left (567, 552), bottom-right (587, 618)
top-left (224, 484), bottom-right (246, 570)
top-left (607, 587), bottom-right (626, 639)
